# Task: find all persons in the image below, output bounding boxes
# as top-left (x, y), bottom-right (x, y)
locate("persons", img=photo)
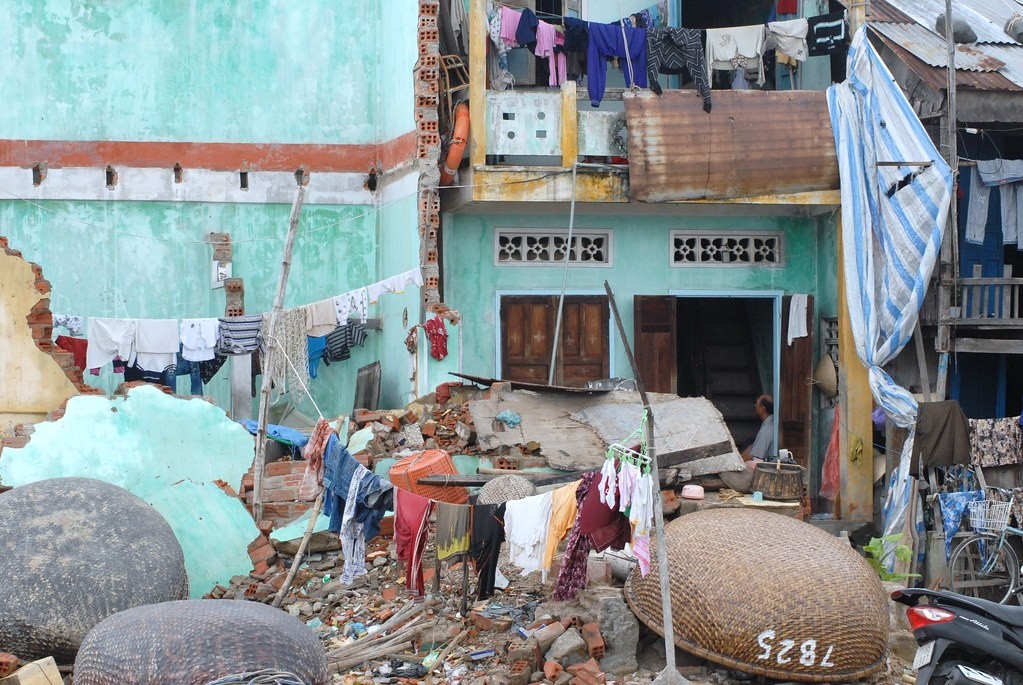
top-left (740, 394), bottom-right (775, 462)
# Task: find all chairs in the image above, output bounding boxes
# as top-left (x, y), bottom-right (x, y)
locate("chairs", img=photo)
top-left (437, 53), bottom-right (470, 124)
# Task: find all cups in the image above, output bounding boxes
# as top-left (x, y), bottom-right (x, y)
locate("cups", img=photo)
top-left (779, 448), bottom-right (793, 460)
top-left (752, 491), bottom-right (762, 501)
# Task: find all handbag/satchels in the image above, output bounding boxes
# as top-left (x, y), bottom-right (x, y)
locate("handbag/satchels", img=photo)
top-left (819, 405), bottom-right (840, 502)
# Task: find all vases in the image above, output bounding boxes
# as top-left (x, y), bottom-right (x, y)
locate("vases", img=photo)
top-left (950, 306), bottom-right (961, 317)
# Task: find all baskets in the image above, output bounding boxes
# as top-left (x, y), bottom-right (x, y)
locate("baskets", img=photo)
top-left (390, 452), bottom-right (468, 505)
top-left (969, 500), bottom-right (1012, 530)
top-left (476, 474), bottom-right (535, 505)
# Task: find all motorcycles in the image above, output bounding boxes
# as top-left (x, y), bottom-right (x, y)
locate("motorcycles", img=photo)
top-left (889, 587), bottom-right (1023, 685)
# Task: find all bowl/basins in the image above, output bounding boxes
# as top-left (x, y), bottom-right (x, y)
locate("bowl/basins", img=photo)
top-left (682, 484), bottom-right (704, 499)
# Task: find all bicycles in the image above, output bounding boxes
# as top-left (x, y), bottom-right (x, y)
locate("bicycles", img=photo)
top-left (947, 485), bottom-right (1023, 605)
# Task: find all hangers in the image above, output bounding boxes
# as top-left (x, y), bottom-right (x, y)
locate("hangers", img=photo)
top-left (603, 409), bottom-right (653, 474)
